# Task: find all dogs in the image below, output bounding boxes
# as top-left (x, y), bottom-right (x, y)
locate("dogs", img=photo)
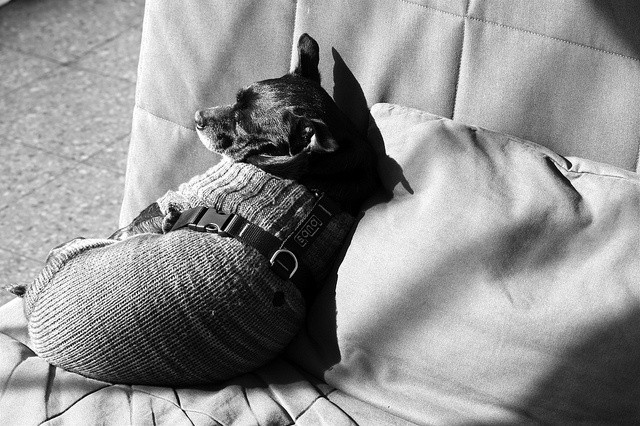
top-left (0, 31), bottom-right (354, 385)
